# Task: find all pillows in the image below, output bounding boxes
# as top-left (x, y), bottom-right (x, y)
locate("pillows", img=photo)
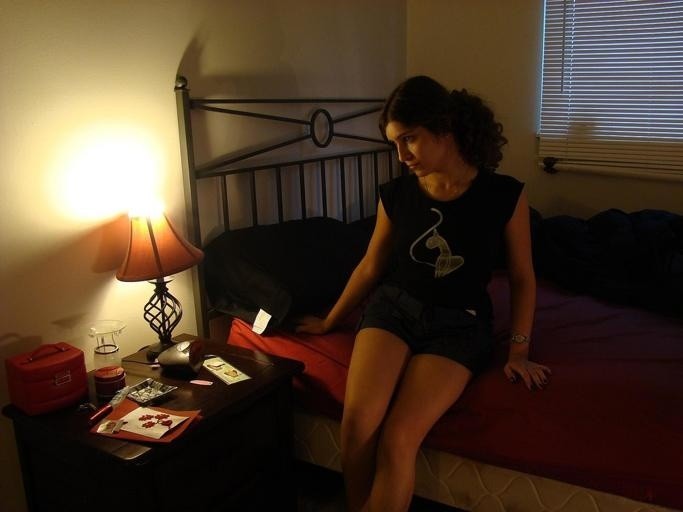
top-left (204, 216), bottom-right (359, 329)
top-left (348, 214), bottom-right (377, 269)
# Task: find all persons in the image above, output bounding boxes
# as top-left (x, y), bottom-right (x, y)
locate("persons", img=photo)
top-left (289, 72), bottom-right (553, 512)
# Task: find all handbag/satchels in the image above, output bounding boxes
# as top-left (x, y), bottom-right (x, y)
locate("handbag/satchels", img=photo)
top-left (5, 338), bottom-right (91, 417)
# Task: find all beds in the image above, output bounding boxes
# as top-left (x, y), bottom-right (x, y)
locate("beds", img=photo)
top-left (173, 75), bottom-right (683, 512)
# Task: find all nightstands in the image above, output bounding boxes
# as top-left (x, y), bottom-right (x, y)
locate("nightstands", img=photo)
top-left (0, 334), bottom-right (305, 512)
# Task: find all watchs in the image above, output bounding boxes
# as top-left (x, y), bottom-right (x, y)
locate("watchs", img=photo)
top-left (507, 333), bottom-right (529, 346)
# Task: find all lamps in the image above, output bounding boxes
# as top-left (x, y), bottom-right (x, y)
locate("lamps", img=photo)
top-left (115, 211), bottom-right (205, 362)
